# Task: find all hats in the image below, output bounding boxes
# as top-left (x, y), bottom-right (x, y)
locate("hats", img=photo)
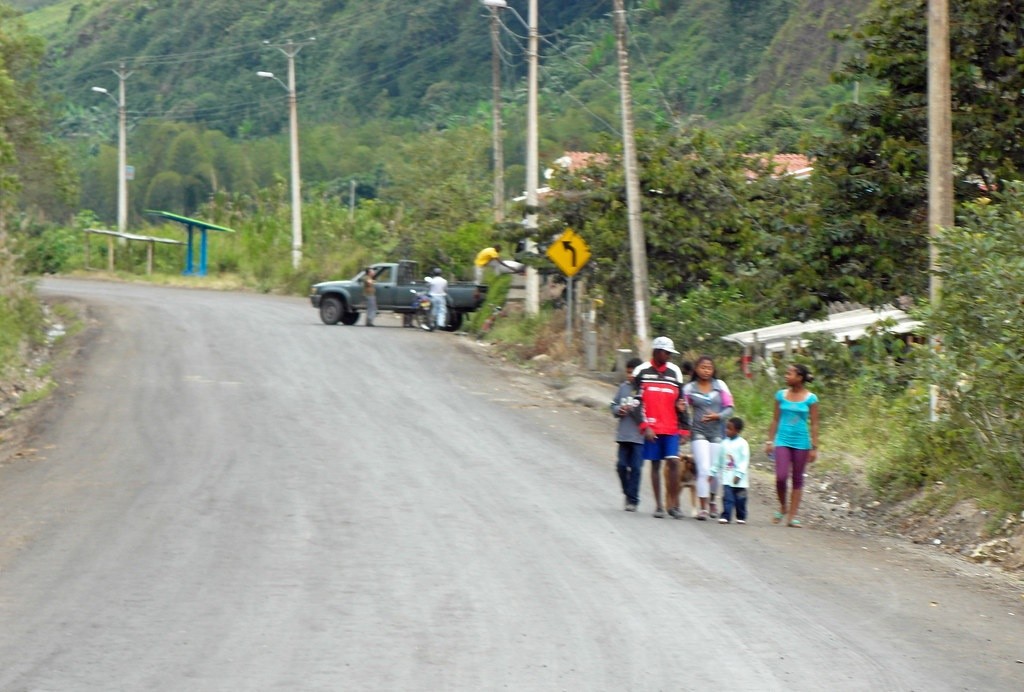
top-left (652, 335), bottom-right (681, 354)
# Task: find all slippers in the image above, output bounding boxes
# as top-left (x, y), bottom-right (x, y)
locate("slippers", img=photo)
top-left (771, 511), bottom-right (786, 525)
top-left (787, 518), bottom-right (802, 529)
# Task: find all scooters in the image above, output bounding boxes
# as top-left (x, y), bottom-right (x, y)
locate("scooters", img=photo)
top-left (410, 290), bottom-right (434, 332)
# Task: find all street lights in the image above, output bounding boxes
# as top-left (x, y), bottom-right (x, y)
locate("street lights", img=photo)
top-left (482, 0), bottom-right (539, 319)
top-left (257, 71), bottom-right (302, 268)
top-left (90, 86), bottom-right (128, 247)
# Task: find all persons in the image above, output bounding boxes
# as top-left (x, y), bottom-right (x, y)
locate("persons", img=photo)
top-left (766, 365), bottom-right (820, 527)
top-left (363, 268), bottom-right (377, 326)
top-left (633, 336), bottom-right (690, 518)
top-left (426, 268), bottom-right (447, 329)
top-left (610, 358), bottom-right (643, 511)
top-left (709, 417), bottom-right (750, 523)
top-left (683, 357), bottom-right (735, 520)
top-left (473, 245), bottom-right (515, 297)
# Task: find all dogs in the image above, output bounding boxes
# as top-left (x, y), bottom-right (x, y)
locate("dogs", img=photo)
top-left (661, 454), bottom-right (703, 518)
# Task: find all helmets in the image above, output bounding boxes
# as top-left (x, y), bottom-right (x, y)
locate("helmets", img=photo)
top-left (433, 267), bottom-right (442, 274)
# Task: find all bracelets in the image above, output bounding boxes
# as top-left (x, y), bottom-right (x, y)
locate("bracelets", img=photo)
top-left (766, 441), bottom-right (773, 444)
top-left (813, 445), bottom-right (818, 450)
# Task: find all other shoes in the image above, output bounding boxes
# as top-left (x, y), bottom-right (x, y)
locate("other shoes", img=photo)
top-left (736, 518), bottom-right (745, 524)
top-left (718, 516), bottom-right (729, 522)
top-left (653, 506), bottom-right (665, 519)
top-left (711, 501), bottom-right (718, 519)
top-left (668, 507), bottom-right (683, 519)
top-left (697, 509), bottom-right (708, 520)
top-left (625, 496), bottom-right (637, 512)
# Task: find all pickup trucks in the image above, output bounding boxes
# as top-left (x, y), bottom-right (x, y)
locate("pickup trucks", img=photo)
top-left (309, 261), bottom-right (489, 332)
